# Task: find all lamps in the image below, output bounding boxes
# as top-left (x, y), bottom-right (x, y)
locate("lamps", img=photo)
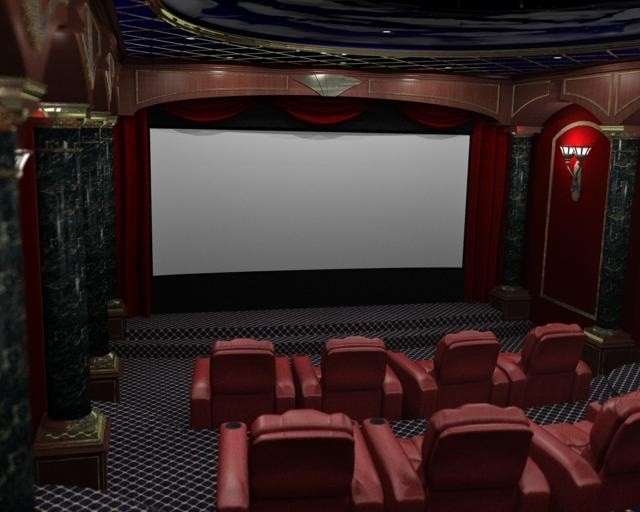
top-left (560, 145), bottom-right (594, 202)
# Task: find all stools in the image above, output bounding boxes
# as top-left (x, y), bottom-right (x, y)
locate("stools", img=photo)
top-left (585, 323), bottom-right (636, 379)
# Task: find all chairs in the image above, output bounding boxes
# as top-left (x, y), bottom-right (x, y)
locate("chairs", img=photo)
top-left (496, 323), bottom-right (590, 404)
top-left (363, 403), bottom-right (550, 511)
top-left (386, 331), bottom-right (509, 414)
top-left (191, 340), bottom-right (296, 431)
top-left (530, 390), bottom-right (638, 512)
top-left (292, 336), bottom-right (404, 423)
top-left (219, 410), bottom-right (384, 510)
